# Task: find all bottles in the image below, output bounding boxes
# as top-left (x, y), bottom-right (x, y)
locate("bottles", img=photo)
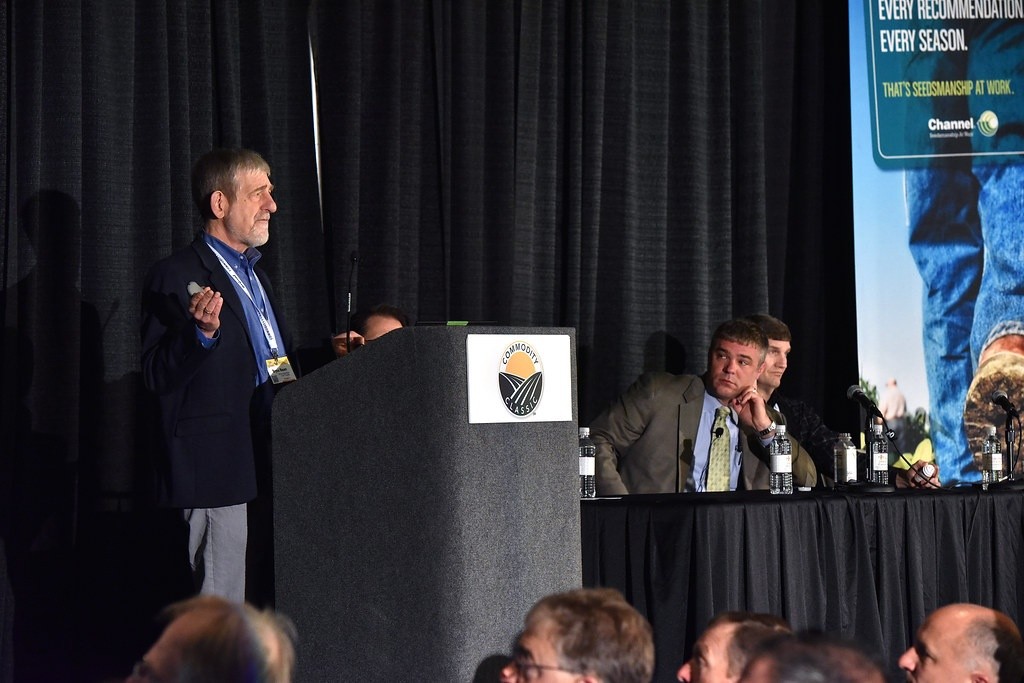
top-left (835, 432), bottom-right (857, 482)
top-left (982, 425), bottom-right (1002, 490)
top-left (868, 424), bottom-right (888, 485)
top-left (577, 427), bottom-right (596, 497)
top-left (769, 425), bottom-right (793, 495)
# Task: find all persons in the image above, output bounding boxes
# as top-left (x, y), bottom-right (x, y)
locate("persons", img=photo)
top-left (877, 378), bottom-right (907, 461)
top-left (677, 612), bottom-right (888, 683)
top-left (127, 595), bottom-right (294, 683)
top-left (901, 0), bottom-right (1024, 482)
top-left (349, 304), bottom-right (408, 342)
top-left (898, 604), bottom-right (1024, 683)
top-left (749, 312), bottom-right (940, 489)
top-left (588, 319), bottom-right (817, 494)
top-left (501, 588), bottom-right (654, 683)
top-left (139, 146), bottom-right (365, 611)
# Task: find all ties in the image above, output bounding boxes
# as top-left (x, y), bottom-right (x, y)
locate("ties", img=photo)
top-left (705, 406), bottom-right (731, 492)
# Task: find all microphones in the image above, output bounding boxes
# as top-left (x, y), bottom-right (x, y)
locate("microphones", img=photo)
top-left (846, 384), bottom-right (884, 418)
top-left (344, 249), bottom-right (359, 355)
top-left (992, 390), bottom-right (1019, 418)
top-left (715, 427), bottom-right (725, 438)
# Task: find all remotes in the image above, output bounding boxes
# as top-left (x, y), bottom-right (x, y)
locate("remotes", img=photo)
top-left (187, 281), bottom-right (204, 297)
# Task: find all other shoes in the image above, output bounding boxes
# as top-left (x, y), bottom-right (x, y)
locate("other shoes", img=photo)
top-left (964, 334), bottom-right (1024, 481)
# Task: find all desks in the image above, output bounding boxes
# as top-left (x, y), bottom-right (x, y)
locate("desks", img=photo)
top-left (579, 484), bottom-right (1024, 683)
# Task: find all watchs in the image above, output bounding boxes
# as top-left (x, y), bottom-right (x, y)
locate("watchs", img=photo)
top-left (752, 419), bottom-right (777, 436)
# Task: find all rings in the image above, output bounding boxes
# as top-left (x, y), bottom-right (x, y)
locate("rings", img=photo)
top-left (204, 308), bottom-right (211, 313)
top-left (754, 388), bottom-right (756, 392)
